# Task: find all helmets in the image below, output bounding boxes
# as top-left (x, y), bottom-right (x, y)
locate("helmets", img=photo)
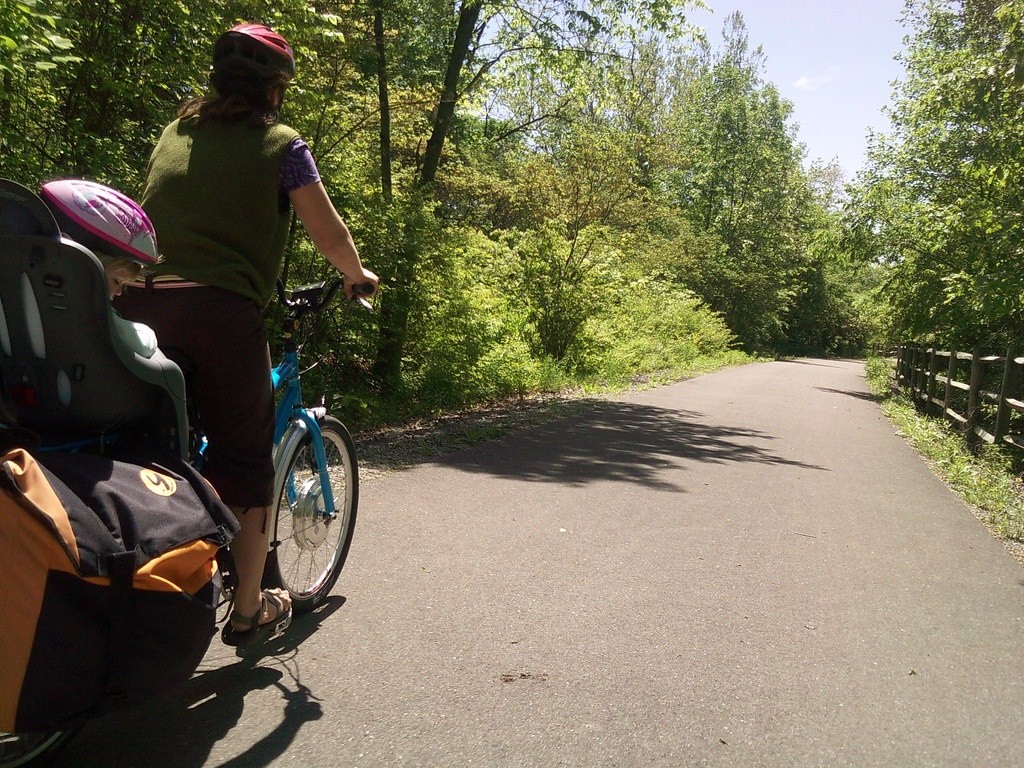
top-left (213, 23), bottom-right (297, 79)
top-left (41, 178), bottom-right (159, 266)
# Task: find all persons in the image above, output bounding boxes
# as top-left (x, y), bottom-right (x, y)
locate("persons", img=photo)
top-left (1, 24), bottom-right (380, 646)
top-left (37, 177), bottom-right (162, 315)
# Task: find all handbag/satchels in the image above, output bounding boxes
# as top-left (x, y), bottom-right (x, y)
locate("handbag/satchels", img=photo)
top-left (1, 434), bottom-right (240, 737)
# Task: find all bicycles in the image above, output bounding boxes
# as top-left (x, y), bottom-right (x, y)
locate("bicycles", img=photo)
top-left (1, 274), bottom-right (359, 768)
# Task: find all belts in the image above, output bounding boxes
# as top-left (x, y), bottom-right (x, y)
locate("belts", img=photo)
top-left (124, 274), bottom-right (208, 288)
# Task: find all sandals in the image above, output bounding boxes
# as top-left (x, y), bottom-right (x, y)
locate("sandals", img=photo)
top-left (221, 589), bottom-right (292, 646)
